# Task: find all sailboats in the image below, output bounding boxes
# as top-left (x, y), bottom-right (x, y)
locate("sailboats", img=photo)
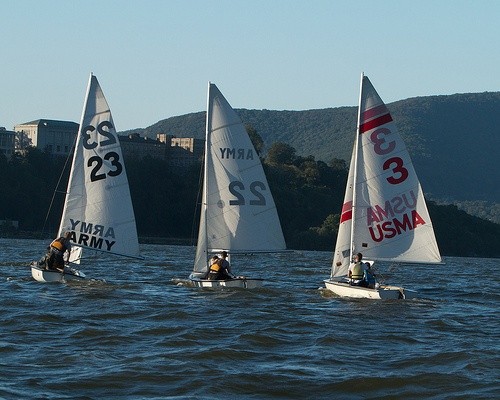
top-left (30, 72), bottom-right (145, 283)
top-left (188, 80), bottom-right (295, 289)
top-left (321, 72), bottom-right (446, 301)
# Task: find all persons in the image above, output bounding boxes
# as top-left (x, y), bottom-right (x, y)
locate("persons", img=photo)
top-left (39, 232), bottom-right (72, 272)
top-left (347, 253), bottom-right (375, 289)
top-left (200, 251), bottom-right (232, 280)
